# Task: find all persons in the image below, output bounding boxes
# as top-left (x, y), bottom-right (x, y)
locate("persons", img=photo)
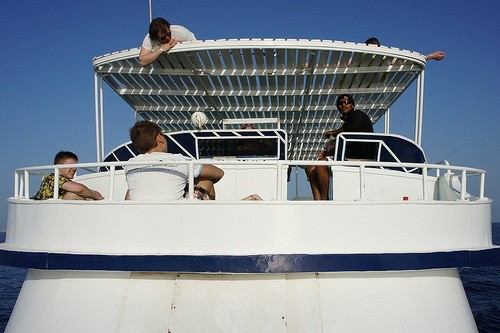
top-left (125, 121), bottom-right (224, 201)
top-left (240, 124), bottom-right (256, 149)
top-left (138, 17), bottom-right (197, 70)
top-left (339, 39), bottom-right (445, 90)
top-left (306, 94), bottom-right (374, 200)
top-left (31, 151), bottom-right (104, 200)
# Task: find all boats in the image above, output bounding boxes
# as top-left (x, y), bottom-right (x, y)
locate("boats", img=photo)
top-left (0, 0), bottom-right (500, 333)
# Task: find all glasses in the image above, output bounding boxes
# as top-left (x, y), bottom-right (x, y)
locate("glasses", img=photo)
top-left (160, 32), bottom-right (171, 41)
top-left (339, 100), bottom-right (352, 104)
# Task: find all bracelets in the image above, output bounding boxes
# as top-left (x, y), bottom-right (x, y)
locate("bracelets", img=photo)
top-left (158, 47), bottom-right (164, 52)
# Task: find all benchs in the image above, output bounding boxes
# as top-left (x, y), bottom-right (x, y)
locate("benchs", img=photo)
top-left (98, 129), bottom-right (288, 202)
top-left (325, 132), bottom-right (426, 201)
